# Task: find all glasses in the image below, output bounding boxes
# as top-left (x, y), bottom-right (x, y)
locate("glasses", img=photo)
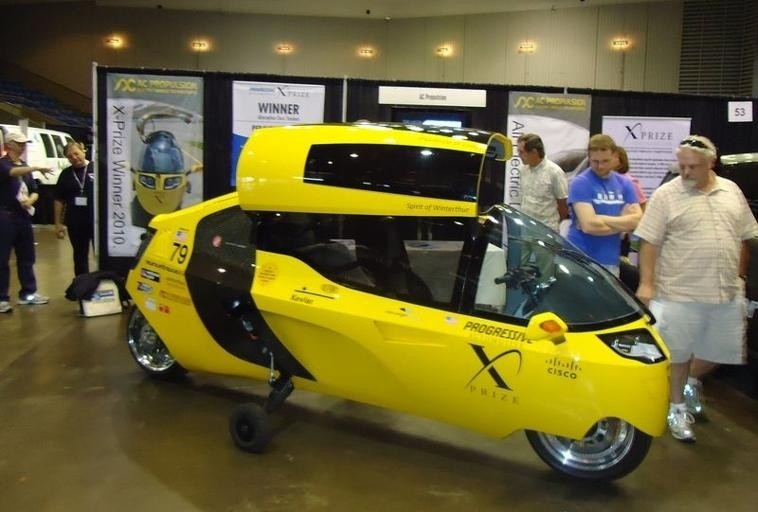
top-left (680, 139), bottom-right (709, 149)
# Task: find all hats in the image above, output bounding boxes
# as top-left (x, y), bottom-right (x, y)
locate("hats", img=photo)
top-left (3, 131), bottom-right (32, 144)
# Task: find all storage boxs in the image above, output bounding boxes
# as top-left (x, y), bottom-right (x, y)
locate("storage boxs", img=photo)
top-left (81, 279), bottom-right (124, 317)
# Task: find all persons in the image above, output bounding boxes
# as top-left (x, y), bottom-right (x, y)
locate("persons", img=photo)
top-left (562, 134), bottom-right (645, 281)
top-left (514, 133), bottom-right (568, 290)
top-left (612, 143), bottom-right (647, 206)
top-left (630, 135), bottom-right (758, 443)
top-left (0, 128), bottom-right (57, 313)
top-left (52, 140), bottom-right (94, 276)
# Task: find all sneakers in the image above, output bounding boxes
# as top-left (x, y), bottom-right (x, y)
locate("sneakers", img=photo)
top-left (665, 406), bottom-right (697, 443)
top-left (683, 384), bottom-right (705, 418)
top-left (0, 301), bottom-right (12, 313)
top-left (16, 289), bottom-right (50, 304)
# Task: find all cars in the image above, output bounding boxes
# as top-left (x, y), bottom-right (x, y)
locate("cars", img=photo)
top-left (545, 148), bottom-right (589, 180)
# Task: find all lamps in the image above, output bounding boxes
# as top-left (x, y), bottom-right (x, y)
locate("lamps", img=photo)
top-left (437, 47), bottom-right (449, 56)
top-left (191, 42), bottom-right (207, 49)
top-left (359, 47), bottom-right (374, 56)
top-left (519, 43), bottom-right (534, 54)
top-left (612, 39), bottom-right (630, 48)
top-left (103, 37), bottom-right (123, 48)
top-left (275, 45), bottom-right (292, 54)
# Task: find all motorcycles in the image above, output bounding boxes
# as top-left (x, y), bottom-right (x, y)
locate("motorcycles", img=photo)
top-left (133, 107), bottom-right (205, 218)
top-left (121, 120), bottom-right (673, 488)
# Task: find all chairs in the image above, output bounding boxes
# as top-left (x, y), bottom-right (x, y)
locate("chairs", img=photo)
top-left (363, 213), bottom-right (437, 305)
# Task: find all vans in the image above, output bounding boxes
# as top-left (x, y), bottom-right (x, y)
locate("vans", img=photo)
top-left (0, 124), bottom-right (86, 189)
